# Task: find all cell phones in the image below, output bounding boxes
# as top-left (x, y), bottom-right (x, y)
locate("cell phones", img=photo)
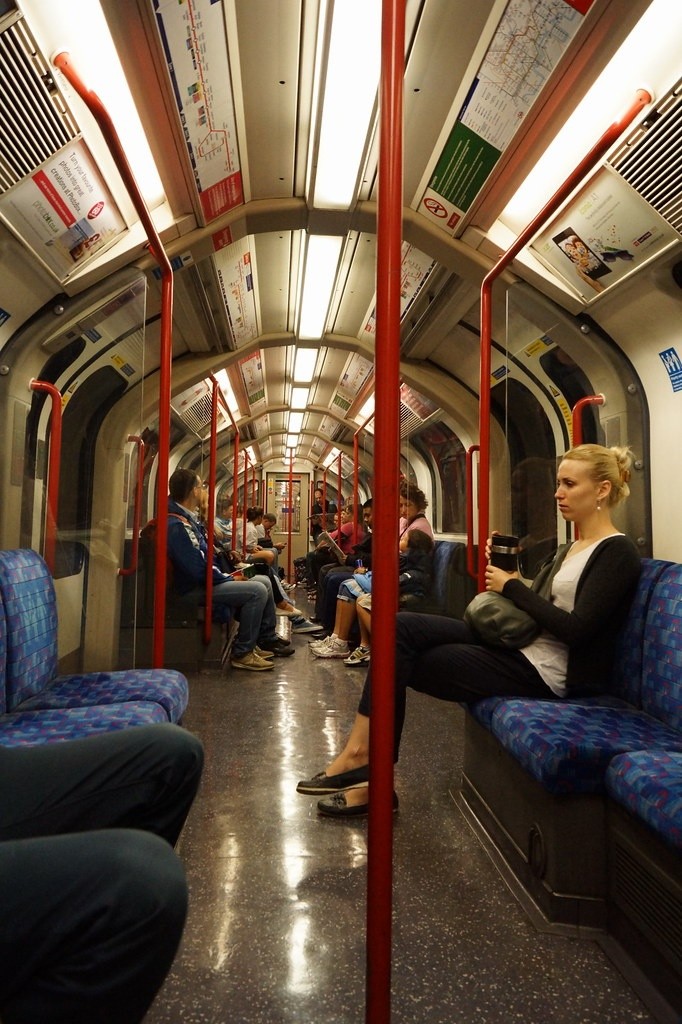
top-left (551, 227), bottom-right (613, 282)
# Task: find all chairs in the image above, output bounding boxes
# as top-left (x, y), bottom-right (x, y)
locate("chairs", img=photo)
top-left (415, 540), bottom-right (682, 849)
top-left (0, 549), bottom-right (190, 753)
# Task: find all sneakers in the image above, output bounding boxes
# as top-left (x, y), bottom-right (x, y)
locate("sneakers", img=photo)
top-left (291, 617), bottom-right (323, 633)
top-left (311, 639), bottom-right (350, 657)
top-left (309, 636), bottom-right (333, 649)
top-left (231, 648), bottom-right (276, 670)
top-left (343, 646), bottom-right (371, 665)
top-left (255, 645), bottom-right (274, 658)
top-left (280, 580), bottom-right (296, 593)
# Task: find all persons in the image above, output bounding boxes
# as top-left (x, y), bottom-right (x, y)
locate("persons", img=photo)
top-left (296, 442), bottom-right (642, 817)
top-left (136, 469), bottom-right (324, 671)
top-left (0, 723), bottom-right (204, 1024)
top-left (292, 488), bottom-right (436, 665)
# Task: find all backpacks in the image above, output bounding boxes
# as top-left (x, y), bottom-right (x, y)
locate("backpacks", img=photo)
top-left (140, 513), bottom-right (189, 585)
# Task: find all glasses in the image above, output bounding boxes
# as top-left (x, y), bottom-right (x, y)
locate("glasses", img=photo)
top-left (197, 484), bottom-right (207, 490)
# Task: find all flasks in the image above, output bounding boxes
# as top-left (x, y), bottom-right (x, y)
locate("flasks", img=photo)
top-left (491, 535), bottom-right (519, 572)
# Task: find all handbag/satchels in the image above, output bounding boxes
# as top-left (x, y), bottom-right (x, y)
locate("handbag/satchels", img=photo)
top-left (462, 542), bottom-right (575, 649)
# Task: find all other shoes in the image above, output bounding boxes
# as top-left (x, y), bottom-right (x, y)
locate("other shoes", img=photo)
top-left (311, 630), bottom-right (332, 640)
top-left (275, 606), bottom-right (302, 616)
top-left (296, 764), bottom-right (369, 795)
top-left (256, 634), bottom-right (295, 657)
top-left (297, 578), bottom-right (307, 584)
top-left (317, 788), bottom-right (398, 817)
